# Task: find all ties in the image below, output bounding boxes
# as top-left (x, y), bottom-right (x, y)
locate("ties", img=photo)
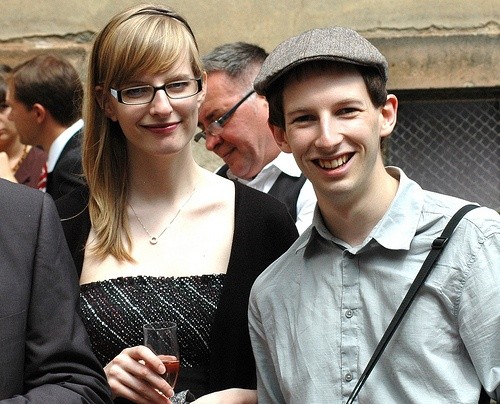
top-left (36, 162), bottom-right (48, 195)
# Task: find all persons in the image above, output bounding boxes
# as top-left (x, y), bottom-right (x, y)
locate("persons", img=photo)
top-left (194, 41), bottom-right (318, 235)
top-left (54, 4), bottom-right (299, 404)
top-left (6, 54), bottom-right (87, 199)
top-left (248, 26), bottom-right (500, 404)
top-left (1, 179), bottom-right (113, 404)
top-left (0, 64), bottom-right (44, 187)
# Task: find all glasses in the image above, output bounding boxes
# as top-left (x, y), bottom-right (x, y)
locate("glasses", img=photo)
top-left (194, 90), bottom-right (256, 146)
top-left (107, 76), bottom-right (206, 105)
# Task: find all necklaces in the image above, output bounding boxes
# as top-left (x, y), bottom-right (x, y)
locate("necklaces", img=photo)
top-left (129, 185), bottom-right (199, 244)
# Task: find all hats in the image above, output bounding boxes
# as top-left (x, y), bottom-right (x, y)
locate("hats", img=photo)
top-left (254, 26), bottom-right (389, 95)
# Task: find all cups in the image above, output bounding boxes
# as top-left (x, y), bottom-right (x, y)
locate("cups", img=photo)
top-left (142, 321), bottom-right (180, 392)
